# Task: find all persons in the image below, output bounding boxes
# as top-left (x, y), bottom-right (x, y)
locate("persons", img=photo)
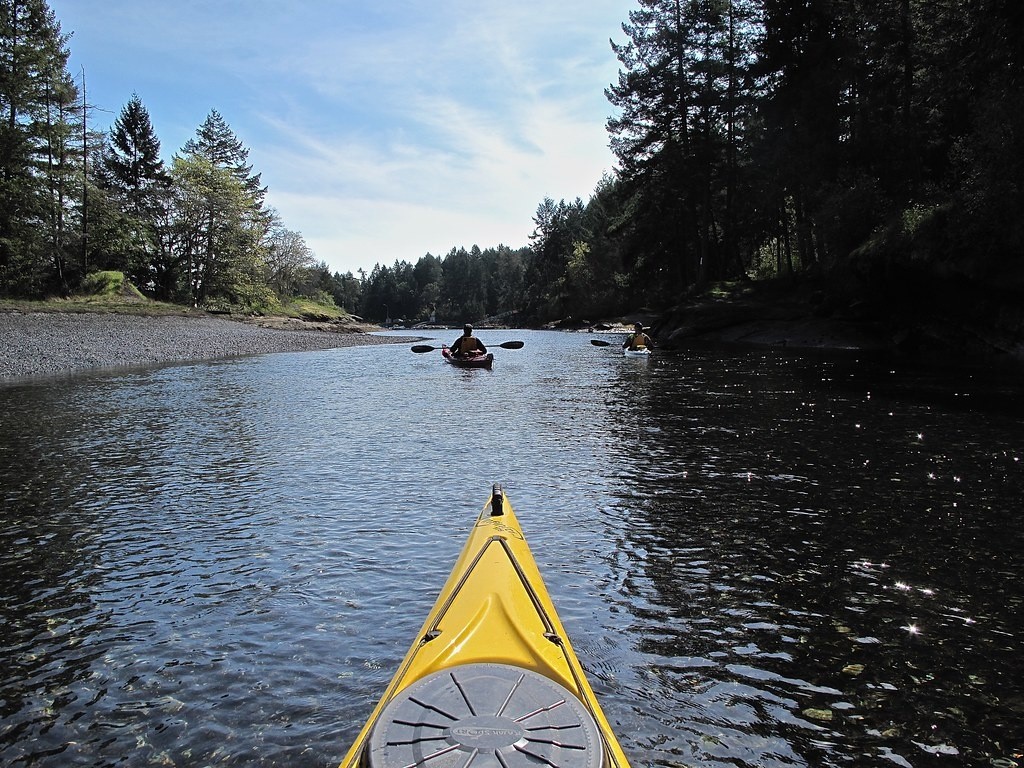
top-left (450, 323), bottom-right (487, 357)
top-left (622, 322), bottom-right (654, 351)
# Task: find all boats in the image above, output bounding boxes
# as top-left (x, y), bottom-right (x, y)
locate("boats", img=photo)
top-left (622, 341), bottom-right (651, 358)
top-left (442, 344), bottom-right (493, 370)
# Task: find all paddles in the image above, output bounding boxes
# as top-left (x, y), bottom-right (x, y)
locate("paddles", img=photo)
top-left (411, 340), bottom-right (524, 354)
top-left (590, 339), bottom-right (681, 351)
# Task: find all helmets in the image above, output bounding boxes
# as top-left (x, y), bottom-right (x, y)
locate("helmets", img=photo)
top-left (464, 324), bottom-right (474, 329)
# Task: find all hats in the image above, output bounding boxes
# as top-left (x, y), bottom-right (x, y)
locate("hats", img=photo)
top-left (635, 322), bottom-right (643, 327)
